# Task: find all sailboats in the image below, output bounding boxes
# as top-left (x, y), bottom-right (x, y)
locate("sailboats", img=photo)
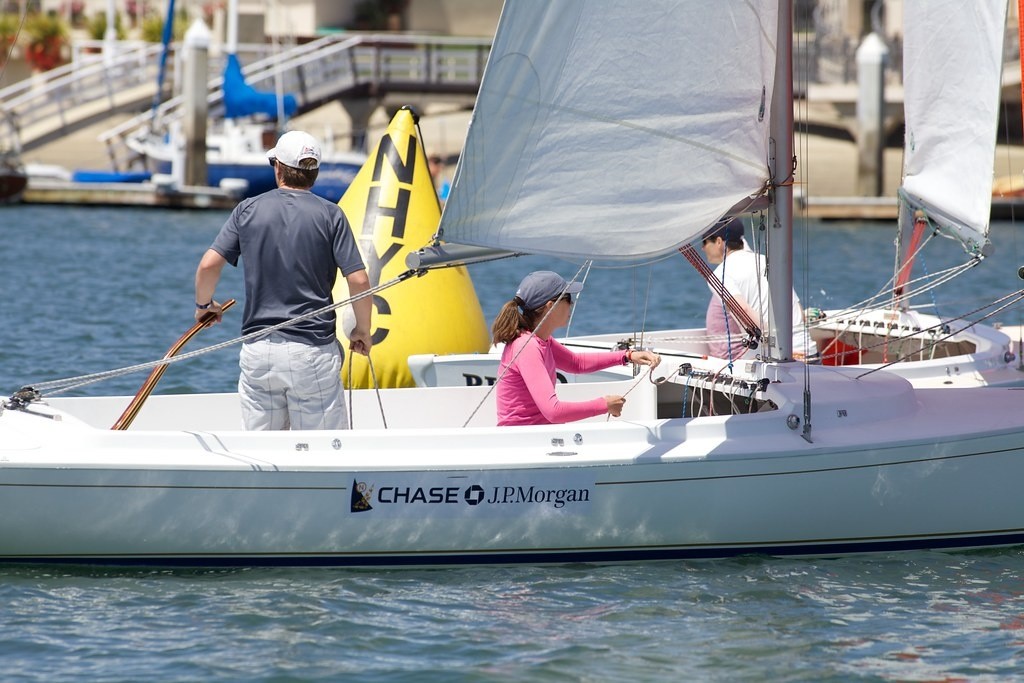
top-left (117, 1), bottom-right (370, 205)
top-left (0, 1), bottom-right (1023, 562)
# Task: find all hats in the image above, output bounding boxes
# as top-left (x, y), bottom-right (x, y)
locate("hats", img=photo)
top-left (266, 131), bottom-right (320, 170)
top-left (703, 217), bottom-right (744, 242)
top-left (516, 271), bottom-right (583, 311)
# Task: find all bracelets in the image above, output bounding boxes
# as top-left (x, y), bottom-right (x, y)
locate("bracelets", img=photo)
top-left (195, 299), bottom-right (213, 309)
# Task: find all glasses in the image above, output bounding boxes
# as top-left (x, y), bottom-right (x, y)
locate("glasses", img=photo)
top-left (703, 237), bottom-right (717, 245)
top-left (535, 293), bottom-right (571, 312)
top-left (269, 157), bottom-right (281, 166)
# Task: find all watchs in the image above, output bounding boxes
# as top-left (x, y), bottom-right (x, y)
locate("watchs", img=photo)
top-left (629, 349), bottom-right (636, 363)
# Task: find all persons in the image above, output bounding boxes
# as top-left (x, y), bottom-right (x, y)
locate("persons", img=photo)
top-left (195, 131), bottom-right (373, 430)
top-left (701, 215), bottom-right (822, 365)
top-left (706, 295), bottom-right (743, 360)
top-left (429, 157), bottom-right (448, 204)
top-left (493, 271), bottom-right (662, 427)
